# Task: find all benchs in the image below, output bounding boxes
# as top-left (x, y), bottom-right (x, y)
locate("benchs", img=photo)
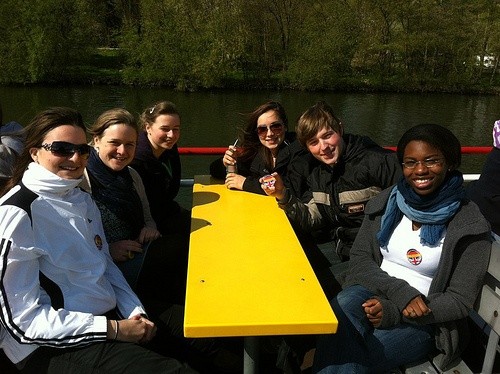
top-left (318, 187), bottom-right (500, 374)
top-left (183, 173), bottom-right (339, 374)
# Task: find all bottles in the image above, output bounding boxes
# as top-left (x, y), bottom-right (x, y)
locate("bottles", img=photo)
top-left (227, 149), bottom-right (238, 190)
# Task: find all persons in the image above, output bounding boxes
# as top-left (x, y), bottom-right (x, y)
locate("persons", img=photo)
top-left (312, 123), bottom-right (494, 374)
top-left (243, 103), bottom-right (402, 374)
top-left (210, 101), bottom-right (297, 195)
top-left (127, 100), bottom-right (192, 239)
top-left (0, 107), bottom-right (222, 374)
top-left (464, 119), bottom-right (500, 235)
top-left (79, 108), bottom-right (187, 305)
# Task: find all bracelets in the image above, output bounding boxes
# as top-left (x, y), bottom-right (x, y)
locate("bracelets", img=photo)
top-left (113, 320), bottom-right (118, 341)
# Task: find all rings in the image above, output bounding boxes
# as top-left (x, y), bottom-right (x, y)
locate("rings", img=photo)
top-left (409, 311), bottom-right (414, 315)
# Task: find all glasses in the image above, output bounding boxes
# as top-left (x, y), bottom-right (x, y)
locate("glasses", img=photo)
top-left (255, 125), bottom-right (282, 135)
top-left (402, 159), bottom-right (447, 168)
top-left (40, 141), bottom-right (90, 160)
